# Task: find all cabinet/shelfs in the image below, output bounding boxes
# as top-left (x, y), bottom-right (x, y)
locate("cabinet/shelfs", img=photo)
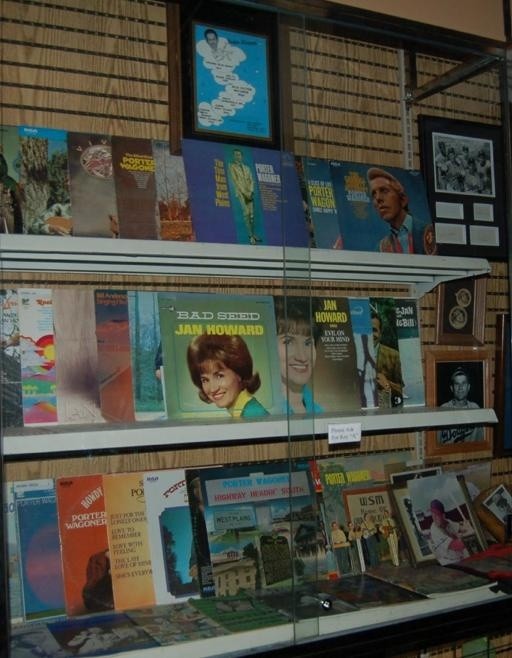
top-left (0, 233), bottom-right (512, 658)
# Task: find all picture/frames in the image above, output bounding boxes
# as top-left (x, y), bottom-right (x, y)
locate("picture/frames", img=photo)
top-left (424, 349), bottom-right (495, 465)
top-left (434, 269), bottom-right (488, 346)
top-left (165, 0), bottom-right (295, 155)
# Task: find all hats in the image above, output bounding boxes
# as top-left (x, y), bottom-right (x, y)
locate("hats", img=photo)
top-left (430, 500), bottom-right (444, 515)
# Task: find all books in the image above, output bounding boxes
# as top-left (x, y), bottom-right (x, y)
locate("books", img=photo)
top-left (0, 124), bottom-right (511, 658)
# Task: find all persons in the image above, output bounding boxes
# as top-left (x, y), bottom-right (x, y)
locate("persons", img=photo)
top-left (204, 29), bottom-right (218, 50)
